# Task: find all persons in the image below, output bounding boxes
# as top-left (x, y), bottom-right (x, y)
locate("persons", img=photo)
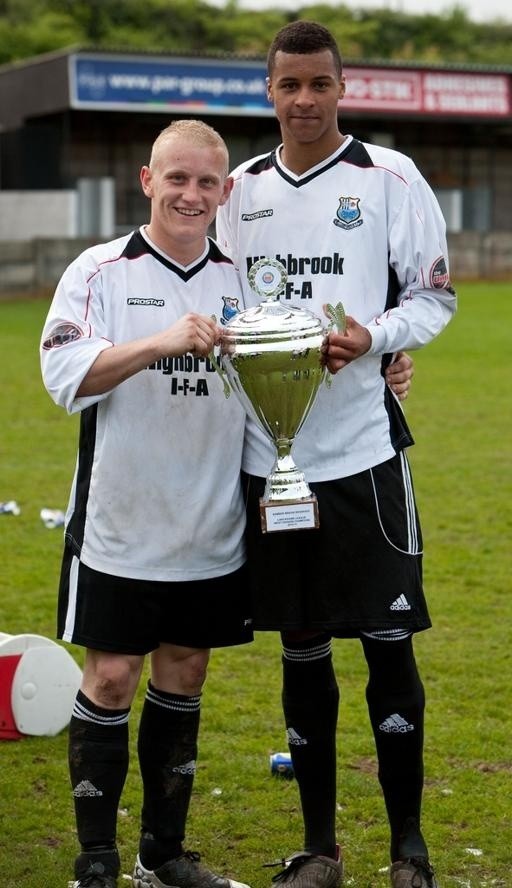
top-left (214, 21), bottom-right (460, 888)
top-left (39, 117), bottom-right (415, 885)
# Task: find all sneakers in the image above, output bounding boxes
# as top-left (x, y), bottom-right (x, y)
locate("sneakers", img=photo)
top-left (392, 857), bottom-right (435, 887)
top-left (133, 847), bottom-right (251, 887)
top-left (262, 843), bottom-right (343, 887)
top-left (73, 847), bottom-right (121, 887)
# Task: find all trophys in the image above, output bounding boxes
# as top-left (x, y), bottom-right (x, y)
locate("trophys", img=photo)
top-left (202, 253), bottom-right (349, 537)
